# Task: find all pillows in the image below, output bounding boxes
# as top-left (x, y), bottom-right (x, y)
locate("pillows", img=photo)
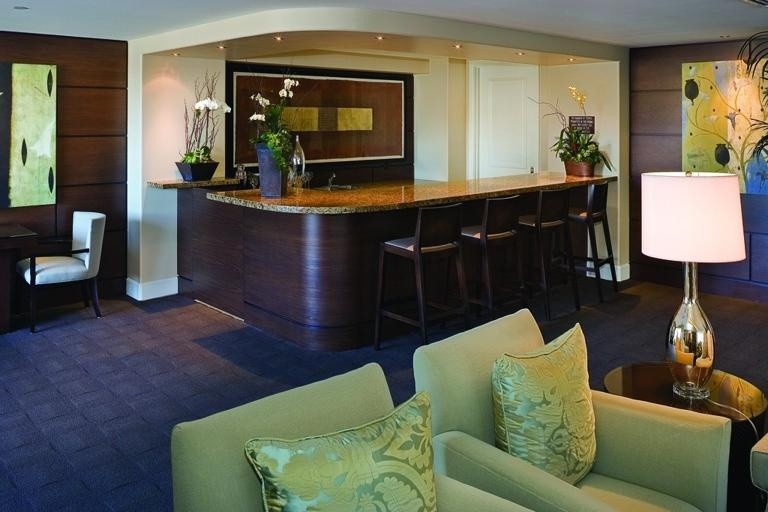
top-left (492, 322), bottom-right (597, 484)
top-left (246, 391), bottom-right (439, 512)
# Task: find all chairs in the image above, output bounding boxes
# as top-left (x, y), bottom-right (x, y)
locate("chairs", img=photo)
top-left (519, 186), bottom-right (581, 319)
top-left (565, 180), bottom-right (619, 302)
top-left (377, 203), bottom-right (470, 344)
top-left (18, 210), bottom-right (107, 334)
top-left (461, 194), bottom-right (530, 320)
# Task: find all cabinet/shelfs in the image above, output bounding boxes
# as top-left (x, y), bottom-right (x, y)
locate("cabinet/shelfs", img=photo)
top-left (180, 172), bottom-right (612, 352)
top-left (1, 29), bottom-right (127, 300)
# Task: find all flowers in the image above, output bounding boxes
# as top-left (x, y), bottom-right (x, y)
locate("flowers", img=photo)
top-left (527, 82), bottom-right (614, 172)
top-left (179, 71), bottom-right (231, 162)
top-left (241, 55), bottom-right (321, 170)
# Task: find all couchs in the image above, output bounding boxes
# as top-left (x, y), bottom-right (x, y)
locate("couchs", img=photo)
top-left (172, 362), bottom-right (533, 511)
top-left (413, 309), bottom-right (731, 512)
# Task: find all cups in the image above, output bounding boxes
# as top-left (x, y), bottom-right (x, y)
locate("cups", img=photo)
top-left (301, 173), bottom-right (307, 192)
top-left (247, 172), bottom-right (260, 188)
top-left (306, 171), bottom-right (313, 192)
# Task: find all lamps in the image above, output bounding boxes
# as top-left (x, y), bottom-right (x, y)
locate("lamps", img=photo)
top-left (642, 172), bottom-right (746, 399)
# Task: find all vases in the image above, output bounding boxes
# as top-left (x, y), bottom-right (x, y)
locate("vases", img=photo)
top-left (176, 163), bottom-right (219, 181)
top-left (564, 160), bottom-right (595, 177)
top-left (254, 141), bottom-right (287, 197)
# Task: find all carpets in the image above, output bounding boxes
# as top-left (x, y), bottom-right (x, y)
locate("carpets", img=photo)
top-left (1, 281), bottom-right (768, 512)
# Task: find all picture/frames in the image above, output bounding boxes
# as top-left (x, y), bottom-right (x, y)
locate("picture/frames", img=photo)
top-left (226, 62), bottom-right (415, 184)
top-left (681, 59), bottom-right (768, 194)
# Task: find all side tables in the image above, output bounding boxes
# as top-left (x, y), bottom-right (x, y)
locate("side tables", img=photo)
top-left (603, 361), bottom-right (768, 422)
